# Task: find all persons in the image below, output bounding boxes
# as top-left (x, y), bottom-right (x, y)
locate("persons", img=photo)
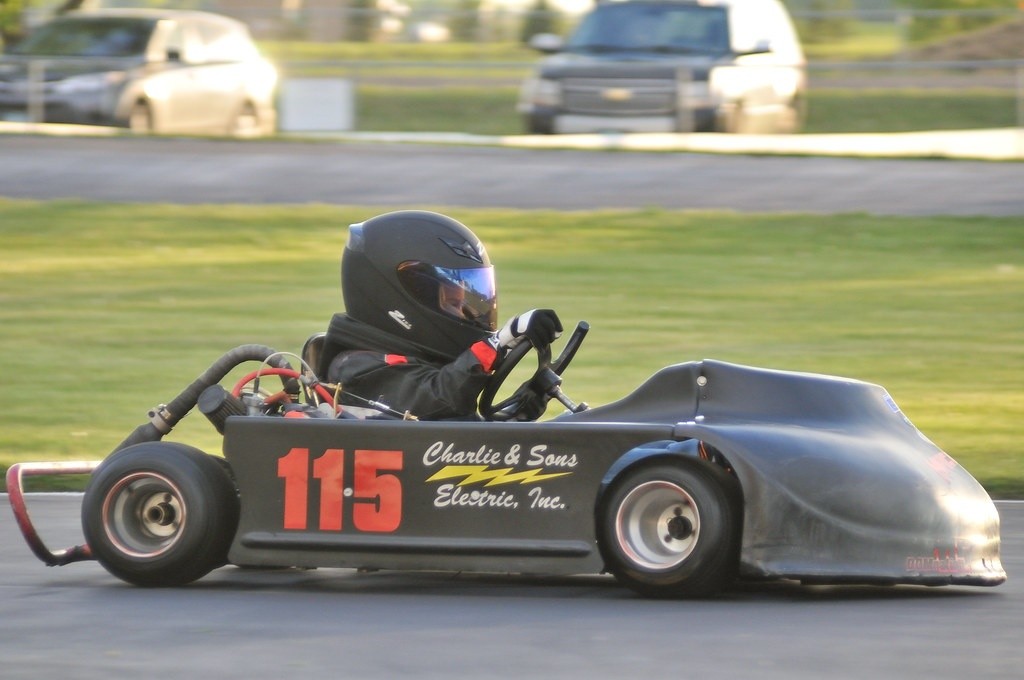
top-left (325, 209), bottom-right (563, 421)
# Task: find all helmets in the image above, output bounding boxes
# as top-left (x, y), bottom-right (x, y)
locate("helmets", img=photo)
top-left (327, 211), bottom-right (498, 369)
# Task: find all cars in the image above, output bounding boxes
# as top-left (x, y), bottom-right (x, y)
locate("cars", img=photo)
top-left (0, 6), bottom-right (280, 139)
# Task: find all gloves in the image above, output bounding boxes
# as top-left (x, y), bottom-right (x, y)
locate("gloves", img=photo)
top-left (482, 308), bottom-right (564, 362)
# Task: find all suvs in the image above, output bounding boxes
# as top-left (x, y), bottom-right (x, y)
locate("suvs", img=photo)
top-left (512, 0), bottom-right (811, 136)
